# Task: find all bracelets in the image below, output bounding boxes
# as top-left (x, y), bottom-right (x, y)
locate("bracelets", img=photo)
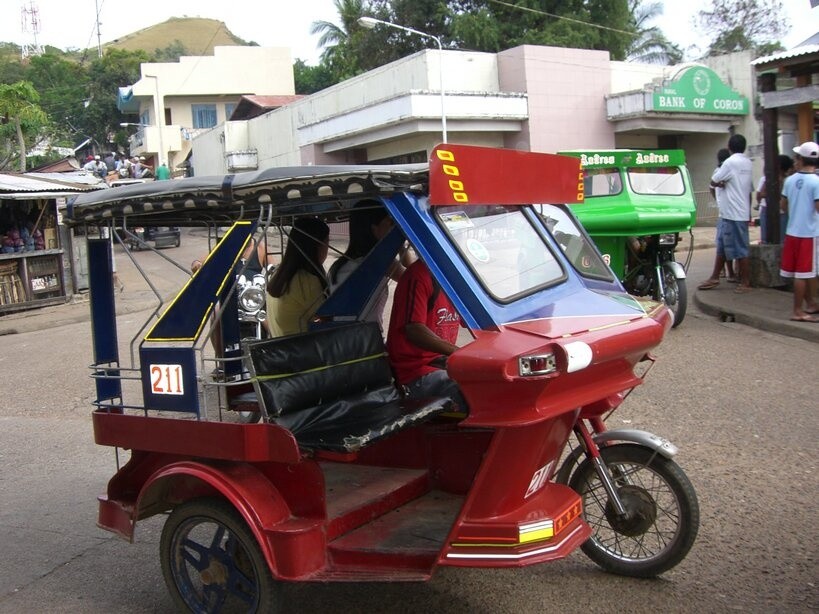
top-left (192, 260), bottom-right (200, 266)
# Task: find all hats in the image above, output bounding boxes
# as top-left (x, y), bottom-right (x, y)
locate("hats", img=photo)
top-left (86, 156), bottom-right (93, 160)
top-left (793, 142), bottom-right (819, 158)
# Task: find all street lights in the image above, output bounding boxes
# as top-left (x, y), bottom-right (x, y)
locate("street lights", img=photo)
top-left (356, 16), bottom-right (448, 142)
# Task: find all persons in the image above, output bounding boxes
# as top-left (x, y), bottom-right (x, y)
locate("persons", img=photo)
top-left (697, 134), bottom-right (755, 293)
top-left (327, 200), bottom-right (414, 342)
top-left (79, 150), bottom-right (154, 178)
top-left (707, 142), bottom-right (819, 323)
top-left (387, 228), bottom-right (475, 424)
top-left (629, 169), bottom-right (655, 253)
top-left (191, 230), bottom-right (277, 382)
top-left (153, 162), bottom-right (174, 180)
top-left (266, 216), bottom-right (330, 338)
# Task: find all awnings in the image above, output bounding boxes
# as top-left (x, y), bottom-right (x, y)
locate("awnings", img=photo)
top-left (0, 174), bottom-right (108, 238)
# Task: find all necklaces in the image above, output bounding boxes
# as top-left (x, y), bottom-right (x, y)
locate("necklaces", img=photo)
top-left (242, 247), bottom-right (249, 258)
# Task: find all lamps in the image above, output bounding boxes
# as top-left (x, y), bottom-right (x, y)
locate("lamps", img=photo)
top-left (119, 122), bottom-right (149, 127)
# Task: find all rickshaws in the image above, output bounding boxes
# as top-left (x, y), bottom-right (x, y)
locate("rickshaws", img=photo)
top-left (556, 149), bottom-right (699, 327)
top-left (56, 142), bottom-right (704, 614)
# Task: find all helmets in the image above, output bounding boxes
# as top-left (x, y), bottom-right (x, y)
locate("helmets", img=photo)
top-left (140, 157), bottom-right (145, 161)
top-left (95, 155), bottom-right (100, 159)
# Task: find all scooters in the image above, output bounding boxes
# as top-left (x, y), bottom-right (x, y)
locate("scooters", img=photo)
top-left (92, 166), bottom-right (182, 252)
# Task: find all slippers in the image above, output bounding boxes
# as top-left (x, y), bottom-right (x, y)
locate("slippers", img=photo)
top-left (791, 313), bottom-right (819, 322)
top-left (805, 310), bottom-right (819, 314)
top-left (735, 286), bottom-right (753, 294)
top-left (698, 281), bottom-right (719, 290)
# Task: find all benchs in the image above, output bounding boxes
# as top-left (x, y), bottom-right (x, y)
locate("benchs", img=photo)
top-left (241, 319), bottom-right (456, 454)
top-left (227, 378), bottom-right (261, 413)
